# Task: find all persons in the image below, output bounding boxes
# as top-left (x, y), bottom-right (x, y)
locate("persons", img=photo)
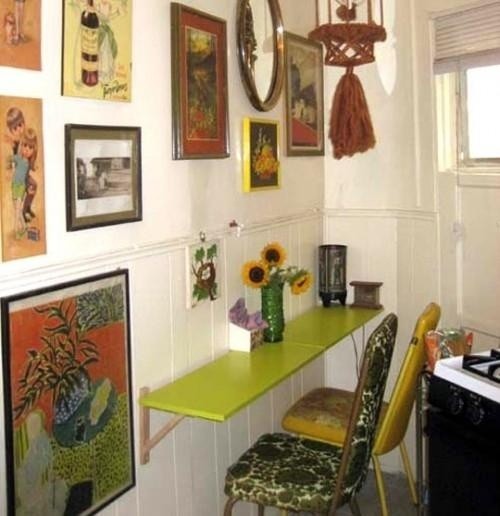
top-left (4, 106), bottom-right (38, 222)
top-left (12, 0), bottom-right (30, 44)
top-left (5, 128), bottom-right (40, 241)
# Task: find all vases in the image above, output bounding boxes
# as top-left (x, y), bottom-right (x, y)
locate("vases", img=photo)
top-left (261, 283), bottom-right (285, 342)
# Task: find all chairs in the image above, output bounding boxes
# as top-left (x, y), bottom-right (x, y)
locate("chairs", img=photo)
top-left (282, 302), bottom-right (441, 516)
top-left (224, 313), bottom-right (398, 515)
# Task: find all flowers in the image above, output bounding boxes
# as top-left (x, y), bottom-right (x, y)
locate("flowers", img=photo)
top-left (241, 242), bottom-right (312, 294)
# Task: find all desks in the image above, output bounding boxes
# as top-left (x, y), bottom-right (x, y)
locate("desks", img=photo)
top-left (285, 301), bottom-right (384, 348)
top-left (138, 341), bottom-right (325, 463)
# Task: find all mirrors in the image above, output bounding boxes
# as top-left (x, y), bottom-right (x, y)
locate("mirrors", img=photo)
top-left (235, 0), bottom-right (286, 111)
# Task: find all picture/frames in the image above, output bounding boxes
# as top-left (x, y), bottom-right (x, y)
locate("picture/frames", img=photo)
top-left (171, 2), bottom-right (231, 160)
top-left (1, 269), bottom-right (136, 516)
top-left (64, 123), bottom-right (142, 231)
top-left (244, 116), bottom-right (282, 190)
top-left (283, 31), bottom-right (325, 157)
top-left (186, 237), bottom-right (228, 308)
